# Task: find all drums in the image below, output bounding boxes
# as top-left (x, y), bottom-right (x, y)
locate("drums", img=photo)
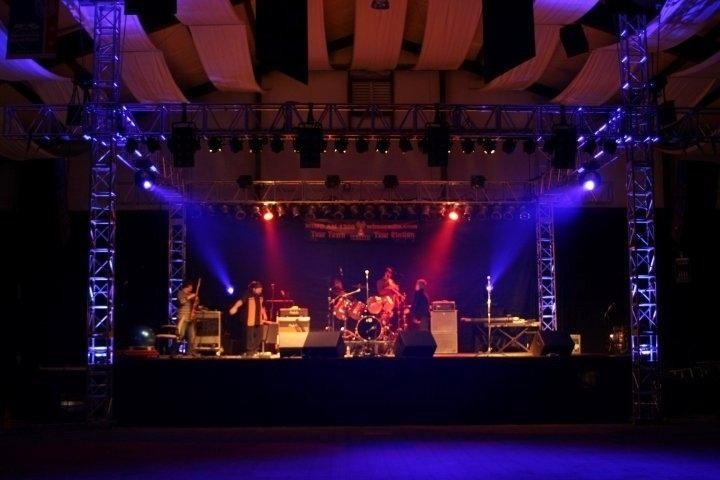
top-left (331, 297), bottom-right (351, 321)
top-left (347, 300), bottom-right (366, 319)
top-left (356, 316), bottom-right (384, 342)
top-left (381, 295), bottom-right (394, 311)
top-left (365, 296), bottom-right (384, 315)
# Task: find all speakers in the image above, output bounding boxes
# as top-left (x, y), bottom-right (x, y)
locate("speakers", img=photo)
top-left (531, 331), bottom-right (575, 356)
top-left (392, 331), bottom-right (437, 357)
top-left (430, 310), bottom-right (458, 354)
top-left (302, 331), bottom-right (345, 357)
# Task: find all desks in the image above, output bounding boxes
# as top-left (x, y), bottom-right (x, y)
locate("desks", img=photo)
top-left (487, 322), bottom-right (540, 352)
top-left (459, 316), bottom-right (514, 353)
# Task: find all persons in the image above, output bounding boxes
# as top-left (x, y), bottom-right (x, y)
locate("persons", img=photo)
top-left (408, 279), bottom-right (431, 329)
top-left (376, 267), bottom-right (398, 333)
top-left (172, 280), bottom-right (199, 355)
top-left (332, 278), bottom-right (365, 331)
top-left (229, 281), bottom-right (268, 355)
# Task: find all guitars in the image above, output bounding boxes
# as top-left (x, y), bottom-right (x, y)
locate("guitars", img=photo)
top-left (190, 278), bottom-right (201, 322)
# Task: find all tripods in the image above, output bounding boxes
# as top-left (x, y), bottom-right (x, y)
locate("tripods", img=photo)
top-left (339, 298), bottom-right (406, 341)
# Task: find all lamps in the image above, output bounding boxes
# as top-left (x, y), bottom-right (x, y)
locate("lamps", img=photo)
top-left (131, 165), bottom-right (155, 194)
top-left (581, 165), bottom-right (601, 194)
top-left (205, 199), bottom-right (537, 224)
top-left (124, 130), bottom-right (601, 156)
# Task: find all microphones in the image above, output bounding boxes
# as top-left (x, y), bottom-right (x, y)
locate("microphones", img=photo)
top-left (487, 275), bottom-right (491, 287)
top-left (365, 269), bottom-right (369, 280)
top-left (281, 290), bottom-right (285, 295)
top-left (271, 284), bottom-right (275, 295)
